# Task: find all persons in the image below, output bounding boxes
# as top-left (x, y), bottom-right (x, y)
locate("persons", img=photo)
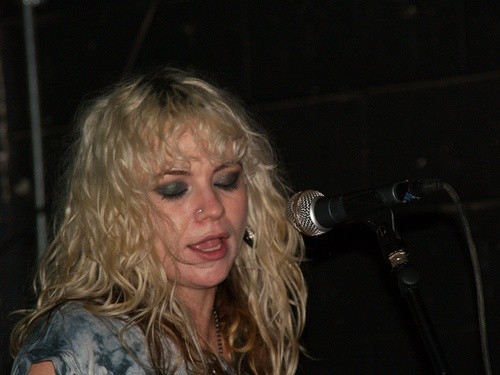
top-left (10, 67), bottom-right (309, 375)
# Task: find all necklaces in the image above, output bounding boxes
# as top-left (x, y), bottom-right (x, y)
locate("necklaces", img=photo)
top-left (192, 307), bottom-right (229, 373)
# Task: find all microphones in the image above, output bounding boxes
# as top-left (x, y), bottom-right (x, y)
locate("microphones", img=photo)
top-left (284, 177), bottom-right (442, 239)
